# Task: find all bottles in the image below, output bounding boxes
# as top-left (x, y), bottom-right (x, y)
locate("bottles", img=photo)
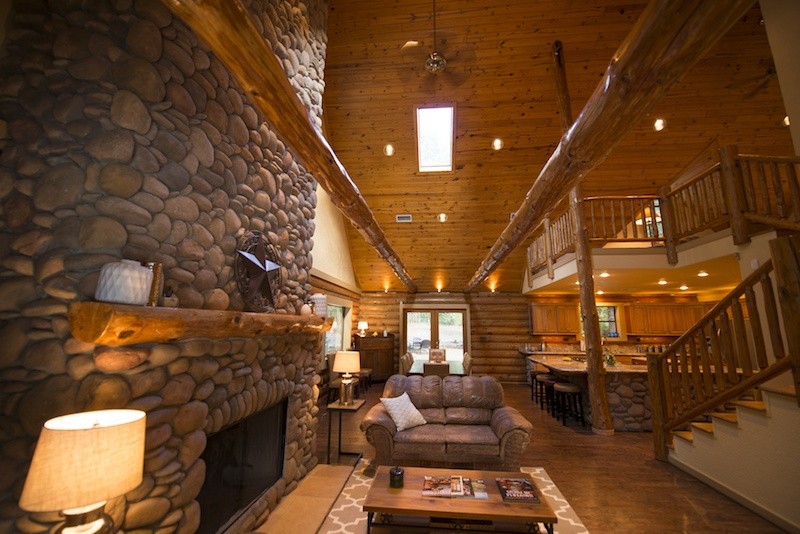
top-left (92, 258), bottom-right (179, 307)
top-left (300, 292), bottom-right (327, 316)
top-left (383, 328), bottom-right (388, 338)
top-left (390, 464), bottom-right (405, 489)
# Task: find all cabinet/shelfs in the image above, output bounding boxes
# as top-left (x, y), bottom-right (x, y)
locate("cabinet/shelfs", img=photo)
top-left (354, 332), bottom-right (395, 381)
top-left (528, 303), bottom-right (582, 335)
top-left (626, 299), bottom-right (750, 336)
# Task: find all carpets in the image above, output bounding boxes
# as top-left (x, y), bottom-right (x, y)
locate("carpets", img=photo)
top-left (317, 457), bottom-right (590, 534)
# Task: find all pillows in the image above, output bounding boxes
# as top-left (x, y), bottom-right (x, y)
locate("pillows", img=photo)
top-left (379, 392), bottom-right (427, 432)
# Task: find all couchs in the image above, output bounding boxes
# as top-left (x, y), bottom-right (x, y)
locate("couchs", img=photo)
top-left (360, 374), bottom-right (535, 472)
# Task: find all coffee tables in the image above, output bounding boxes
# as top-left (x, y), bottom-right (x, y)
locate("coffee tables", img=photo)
top-left (362, 465), bottom-right (558, 534)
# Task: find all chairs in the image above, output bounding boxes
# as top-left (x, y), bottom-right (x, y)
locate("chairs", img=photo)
top-left (401, 349), bottom-right (474, 377)
top-left (326, 353), bottom-right (373, 405)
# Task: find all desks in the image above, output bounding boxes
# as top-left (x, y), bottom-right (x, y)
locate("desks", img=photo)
top-left (407, 360), bottom-right (468, 376)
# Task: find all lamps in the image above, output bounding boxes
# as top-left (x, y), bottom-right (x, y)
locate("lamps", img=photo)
top-left (18, 409), bottom-right (146, 534)
top-left (357, 322), bottom-right (368, 337)
top-left (635, 206), bottom-right (660, 247)
top-left (332, 351), bottom-right (360, 406)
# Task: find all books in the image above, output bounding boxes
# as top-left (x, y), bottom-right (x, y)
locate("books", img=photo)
top-left (495, 476), bottom-right (541, 504)
top-left (421, 474), bottom-right (488, 500)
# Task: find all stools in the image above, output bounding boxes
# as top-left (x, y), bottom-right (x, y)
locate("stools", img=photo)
top-left (530, 371), bottom-right (586, 428)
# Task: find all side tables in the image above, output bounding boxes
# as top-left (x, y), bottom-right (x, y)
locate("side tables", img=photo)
top-left (326, 399), bottom-right (367, 467)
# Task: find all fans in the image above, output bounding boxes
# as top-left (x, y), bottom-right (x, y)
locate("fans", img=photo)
top-left (396, 0), bottom-right (475, 94)
top-left (725, 58), bottom-right (777, 100)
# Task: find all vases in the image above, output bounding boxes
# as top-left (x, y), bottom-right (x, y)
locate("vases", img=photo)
top-left (95, 259), bottom-right (154, 305)
